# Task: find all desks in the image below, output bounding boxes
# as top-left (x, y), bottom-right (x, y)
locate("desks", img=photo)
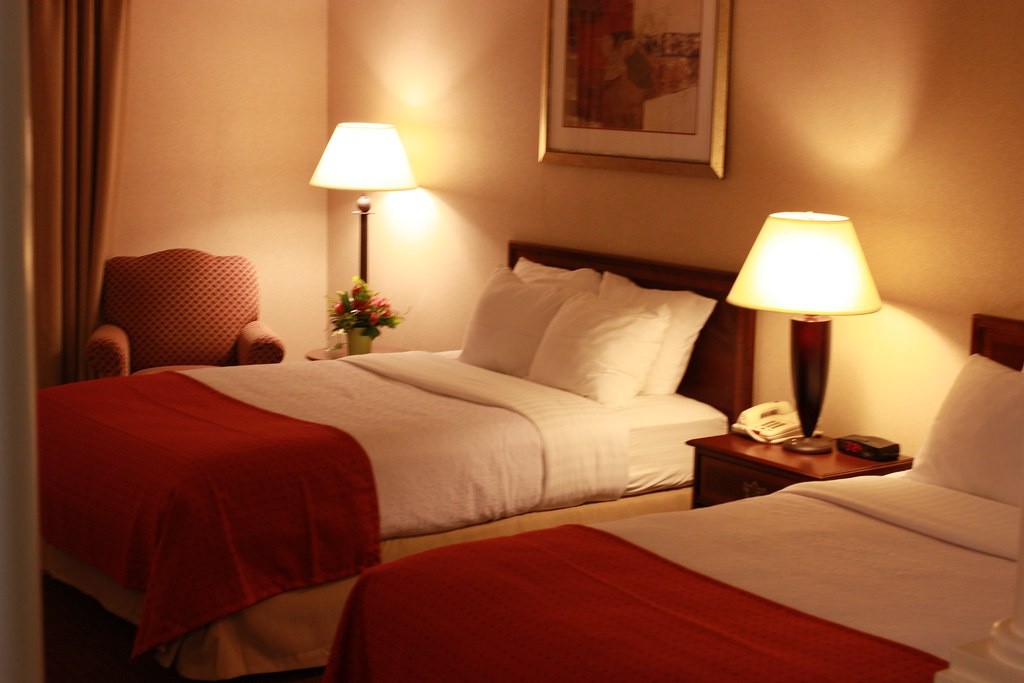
top-left (306, 344), bottom-right (406, 361)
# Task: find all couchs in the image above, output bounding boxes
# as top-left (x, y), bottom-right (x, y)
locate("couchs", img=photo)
top-left (84, 249), bottom-right (285, 382)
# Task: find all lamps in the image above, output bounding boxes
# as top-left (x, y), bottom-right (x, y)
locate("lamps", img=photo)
top-left (308, 122), bottom-right (420, 283)
top-left (725, 210), bottom-right (883, 454)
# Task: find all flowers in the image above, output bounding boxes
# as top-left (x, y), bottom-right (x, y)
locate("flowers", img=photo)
top-left (327, 275), bottom-right (404, 340)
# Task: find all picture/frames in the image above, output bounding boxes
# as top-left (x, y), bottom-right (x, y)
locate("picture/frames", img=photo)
top-left (537, 0), bottom-right (734, 180)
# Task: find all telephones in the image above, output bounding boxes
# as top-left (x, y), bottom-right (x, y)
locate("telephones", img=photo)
top-left (732, 400), bottom-right (823, 441)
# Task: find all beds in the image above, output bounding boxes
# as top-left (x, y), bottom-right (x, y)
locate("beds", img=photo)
top-left (320, 313), bottom-right (1024, 683)
top-left (38, 239), bottom-right (759, 683)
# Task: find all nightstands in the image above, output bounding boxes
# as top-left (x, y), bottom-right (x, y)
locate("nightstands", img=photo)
top-left (686, 432), bottom-right (914, 510)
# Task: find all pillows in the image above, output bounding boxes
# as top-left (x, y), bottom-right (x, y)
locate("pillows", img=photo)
top-left (455, 263), bottom-right (580, 377)
top-left (908, 353), bottom-right (1023, 507)
top-left (527, 288), bottom-right (673, 409)
top-left (513, 256), bottom-right (601, 295)
top-left (598, 271), bottom-right (719, 396)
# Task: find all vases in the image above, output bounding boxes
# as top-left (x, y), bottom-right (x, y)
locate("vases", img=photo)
top-left (346, 328), bottom-right (372, 355)
